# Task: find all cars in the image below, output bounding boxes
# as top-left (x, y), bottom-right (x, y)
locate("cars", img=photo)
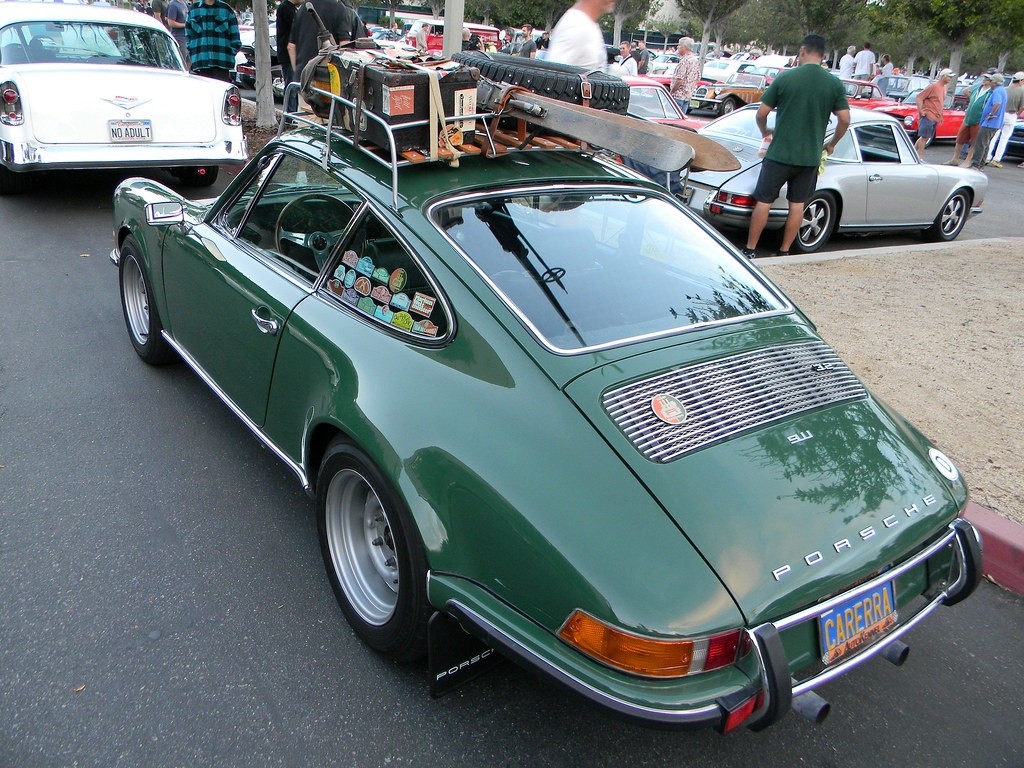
top-left (0, 0), bottom-right (251, 190)
top-left (227, 11), bottom-right (285, 89)
top-left (364, 6), bottom-right (1024, 173)
top-left (681, 98), bottom-right (988, 253)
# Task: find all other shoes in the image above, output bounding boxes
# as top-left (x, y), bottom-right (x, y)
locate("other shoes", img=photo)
top-left (943, 160), bottom-right (957, 166)
top-left (1017, 162), bottom-right (1024, 168)
top-left (767, 250), bottom-right (790, 257)
top-left (992, 159), bottom-right (1004, 168)
top-left (973, 164), bottom-right (986, 171)
top-left (737, 246), bottom-right (754, 259)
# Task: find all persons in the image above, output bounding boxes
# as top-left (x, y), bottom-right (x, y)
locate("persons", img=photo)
top-left (276, 0), bottom-right (367, 113)
top-left (545, 0), bottom-right (616, 74)
top-left (461, 24), bottom-right (550, 59)
top-left (607, 37), bottom-right (766, 114)
top-left (740, 34), bottom-right (850, 259)
top-left (821, 41), bottom-right (1024, 172)
top-left (784, 58), bottom-right (793, 67)
top-left (361, 20), bottom-right (429, 53)
top-left (118, 0), bottom-right (251, 83)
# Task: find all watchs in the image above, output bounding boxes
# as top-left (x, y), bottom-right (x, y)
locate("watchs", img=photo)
top-left (989, 113), bottom-right (993, 116)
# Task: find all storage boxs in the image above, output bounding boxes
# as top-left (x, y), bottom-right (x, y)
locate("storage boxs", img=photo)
top-left (330, 45), bottom-right (477, 152)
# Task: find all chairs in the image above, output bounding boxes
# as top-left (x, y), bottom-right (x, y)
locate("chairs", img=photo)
top-left (523, 225), bottom-right (597, 273)
top-left (365, 238), bottom-right (430, 289)
top-left (2, 46), bottom-right (56, 66)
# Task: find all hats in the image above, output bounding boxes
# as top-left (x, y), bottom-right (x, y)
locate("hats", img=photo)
top-left (981, 71), bottom-right (994, 80)
top-left (939, 67), bottom-right (956, 78)
top-left (1010, 71), bottom-right (1024, 81)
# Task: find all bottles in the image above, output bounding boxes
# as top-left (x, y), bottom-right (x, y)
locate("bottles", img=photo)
top-left (758, 134), bottom-right (773, 156)
top-left (818, 148), bottom-right (827, 175)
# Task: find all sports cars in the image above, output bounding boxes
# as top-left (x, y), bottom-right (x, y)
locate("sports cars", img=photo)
top-left (110, 72), bottom-right (987, 735)
